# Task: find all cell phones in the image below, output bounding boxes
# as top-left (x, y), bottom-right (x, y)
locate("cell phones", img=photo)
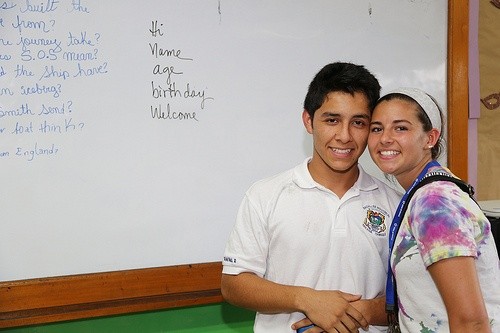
top-left (297, 324), bottom-right (315, 333)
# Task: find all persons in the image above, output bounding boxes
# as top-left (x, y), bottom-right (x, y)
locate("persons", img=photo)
top-left (220, 62), bottom-right (405, 333)
top-left (366, 87), bottom-right (500, 333)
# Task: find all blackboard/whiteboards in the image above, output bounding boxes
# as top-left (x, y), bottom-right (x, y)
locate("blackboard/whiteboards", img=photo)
top-left (0, 0), bottom-right (469, 331)
